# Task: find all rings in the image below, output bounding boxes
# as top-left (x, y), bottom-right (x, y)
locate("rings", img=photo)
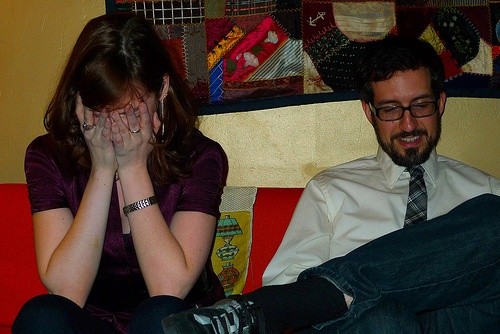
top-left (82, 123), bottom-right (93, 131)
top-left (130, 128), bottom-right (143, 134)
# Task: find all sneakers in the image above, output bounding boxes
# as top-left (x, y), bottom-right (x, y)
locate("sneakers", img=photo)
top-left (161, 295), bottom-right (256, 334)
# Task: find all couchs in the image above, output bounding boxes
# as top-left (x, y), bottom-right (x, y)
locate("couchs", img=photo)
top-left (0, 183), bottom-right (304, 334)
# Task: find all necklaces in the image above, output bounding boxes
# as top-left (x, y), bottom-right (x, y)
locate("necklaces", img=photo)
top-left (113, 170), bottom-right (121, 183)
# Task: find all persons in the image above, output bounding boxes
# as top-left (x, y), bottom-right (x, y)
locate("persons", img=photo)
top-left (10, 12), bottom-right (228, 334)
top-left (160, 34), bottom-right (500, 334)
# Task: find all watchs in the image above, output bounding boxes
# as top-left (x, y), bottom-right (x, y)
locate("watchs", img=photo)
top-left (122, 194), bottom-right (162, 217)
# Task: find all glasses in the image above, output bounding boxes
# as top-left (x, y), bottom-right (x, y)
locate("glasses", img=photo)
top-left (365, 91), bottom-right (440, 122)
